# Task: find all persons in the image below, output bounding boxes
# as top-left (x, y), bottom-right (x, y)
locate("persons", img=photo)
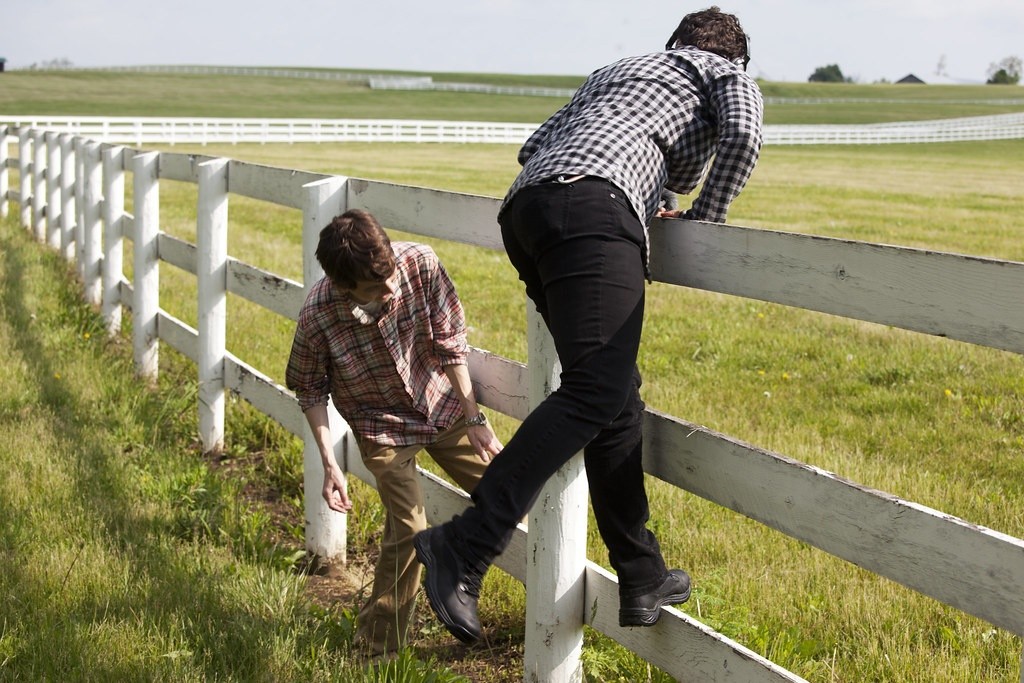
top-left (414, 4), bottom-right (765, 644)
top-left (286, 209), bottom-right (526, 665)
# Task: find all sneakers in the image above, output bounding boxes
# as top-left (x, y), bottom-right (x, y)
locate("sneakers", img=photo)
top-left (618, 569), bottom-right (692, 628)
top-left (412, 525), bottom-right (482, 647)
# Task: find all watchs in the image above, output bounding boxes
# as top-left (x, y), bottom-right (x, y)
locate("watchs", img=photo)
top-left (466, 412), bottom-right (487, 426)
